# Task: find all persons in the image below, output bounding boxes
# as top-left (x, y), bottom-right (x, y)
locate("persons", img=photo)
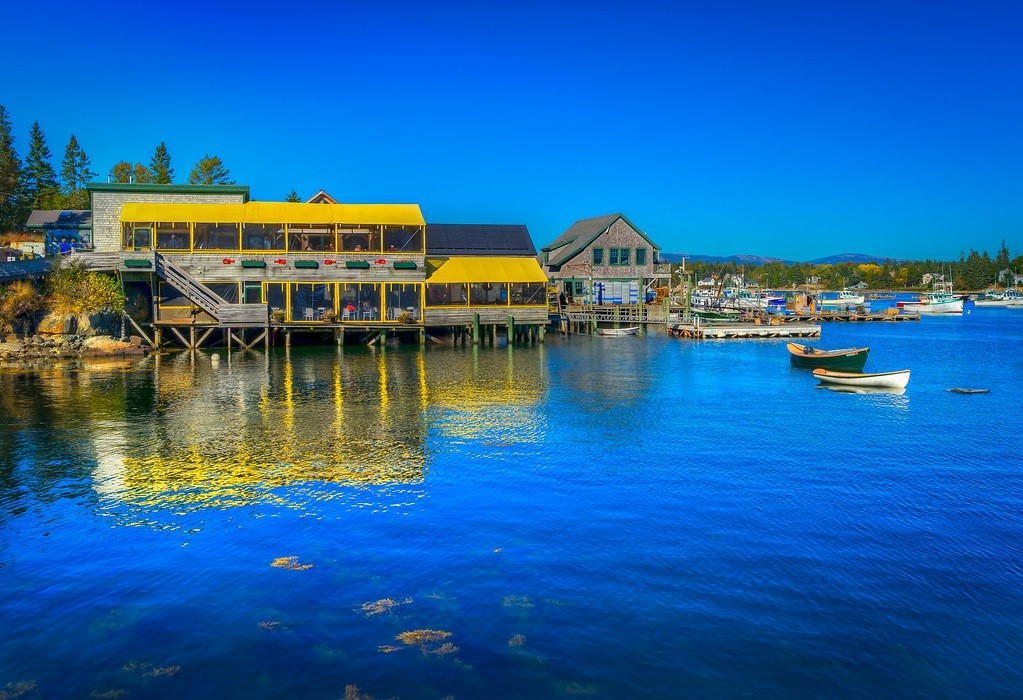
top-left (306, 244), bottom-right (313, 250)
top-left (389, 245), bottom-right (398, 251)
top-left (59, 238), bottom-right (78, 256)
top-left (354, 244), bottom-right (362, 251)
top-left (346, 302), bottom-right (357, 319)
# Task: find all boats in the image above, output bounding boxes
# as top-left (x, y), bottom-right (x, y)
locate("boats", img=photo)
top-left (974, 266), bottom-right (1023, 306)
top-left (815, 382), bottom-right (907, 395)
top-left (787, 342), bottom-right (870, 372)
top-left (813, 368), bottom-right (911, 387)
top-left (897, 258), bottom-right (970, 313)
top-left (691, 278), bottom-right (895, 315)
top-left (594, 327), bottom-right (640, 335)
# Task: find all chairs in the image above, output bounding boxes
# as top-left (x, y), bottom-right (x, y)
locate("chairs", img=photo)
top-left (299, 307), bottom-right (420, 321)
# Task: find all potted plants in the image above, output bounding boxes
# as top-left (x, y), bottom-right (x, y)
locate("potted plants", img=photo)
top-left (396, 310), bottom-right (418, 324)
top-left (269, 309), bottom-right (286, 323)
top-left (322, 307), bottom-right (339, 322)
